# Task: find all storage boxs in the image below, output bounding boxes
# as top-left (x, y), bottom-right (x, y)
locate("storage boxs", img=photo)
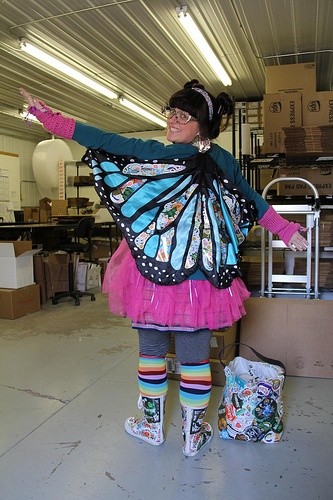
top-left (23, 196), bottom-right (68, 223)
top-left (0, 240), bottom-right (100, 320)
top-left (161, 326), bottom-right (237, 386)
top-left (222, 62), bottom-right (333, 196)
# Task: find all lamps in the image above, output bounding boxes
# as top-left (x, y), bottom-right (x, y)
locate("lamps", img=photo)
top-left (176, 4), bottom-right (233, 87)
top-left (119, 94), bottom-right (167, 129)
top-left (18, 37), bottom-right (119, 100)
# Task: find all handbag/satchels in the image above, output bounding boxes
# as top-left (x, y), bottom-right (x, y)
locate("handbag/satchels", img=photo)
top-left (219, 341), bottom-right (288, 444)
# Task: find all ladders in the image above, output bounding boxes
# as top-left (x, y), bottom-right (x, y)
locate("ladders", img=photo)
top-left (259, 177), bottom-right (322, 300)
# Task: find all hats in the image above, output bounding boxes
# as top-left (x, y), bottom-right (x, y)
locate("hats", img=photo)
top-left (169, 79), bottom-right (232, 138)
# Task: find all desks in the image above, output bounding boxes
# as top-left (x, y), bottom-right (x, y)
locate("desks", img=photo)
top-left (0, 222), bottom-right (68, 251)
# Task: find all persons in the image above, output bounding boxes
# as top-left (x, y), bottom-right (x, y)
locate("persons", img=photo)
top-left (19, 77), bottom-right (309, 458)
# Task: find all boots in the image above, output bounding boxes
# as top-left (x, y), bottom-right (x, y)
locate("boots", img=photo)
top-left (124, 395), bottom-right (167, 446)
top-left (179, 405), bottom-right (214, 457)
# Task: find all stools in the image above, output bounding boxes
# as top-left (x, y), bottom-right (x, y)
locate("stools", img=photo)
top-left (52, 215), bottom-right (97, 306)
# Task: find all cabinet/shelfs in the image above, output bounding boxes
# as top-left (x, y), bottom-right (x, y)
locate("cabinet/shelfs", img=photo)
top-left (65, 160), bottom-right (124, 259)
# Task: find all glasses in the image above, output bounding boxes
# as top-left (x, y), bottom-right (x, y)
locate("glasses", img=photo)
top-left (160, 105), bottom-right (197, 125)
